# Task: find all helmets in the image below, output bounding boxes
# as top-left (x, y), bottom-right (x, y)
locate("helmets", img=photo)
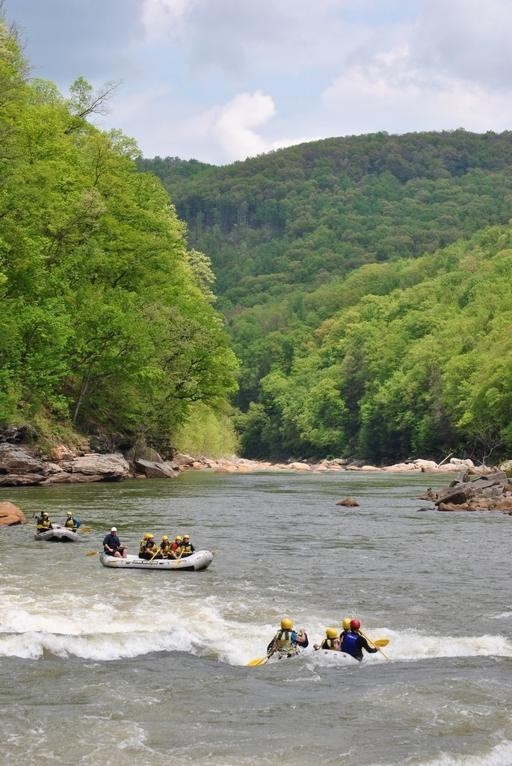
top-left (350, 620), bottom-right (362, 632)
top-left (325, 628), bottom-right (338, 640)
top-left (176, 535), bottom-right (182, 540)
top-left (280, 618), bottom-right (295, 632)
top-left (66, 512), bottom-right (73, 516)
top-left (342, 618), bottom-right (351, 630)
top-left (43, 512), bottom-right (49, 516)
top-left (183, 534), bottom-right (189, 539)
top-left (162, 535), bottom-right (168, 541)
top-left (144, 533), bottom-right (154, 541)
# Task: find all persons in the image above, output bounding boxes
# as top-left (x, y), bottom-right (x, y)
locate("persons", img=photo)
top-left (65, 511), bottom-right (81, 533)
top-left (103, 526), bottom-right (129, 558)
top-left (139, 533), bottom-right (196, 560)
top-left (37, 512), bottom-right (53, 534)
top-left (32, 511), bottom-right (44, 520)
top-left (335, 618), bottom-right (351, 650)
top-left (267, 620), bottom-right (308, 656)
top-left (312, 628), bottom-right (338, 650)
top-left (340, 620), bottom-right (380, 663)
top-left (264, 617), bottom-right (306, 658)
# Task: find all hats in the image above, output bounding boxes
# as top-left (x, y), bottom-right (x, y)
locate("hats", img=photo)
top-left (110, 527), bottom-right (118, 532)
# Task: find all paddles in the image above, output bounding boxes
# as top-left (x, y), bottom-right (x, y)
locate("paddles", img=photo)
top-left (175, 548), bottom-right (186, 567)
top-left (86, 548), bottom-right (123, 556)
top-left (367, 638), bottom-right (389, 646)
top-left (247, 653), bottom-right (270, 667)
top-left (57, 526), bottom-right (91, 532)
top-left (37, 524), bottom-right (51, 530)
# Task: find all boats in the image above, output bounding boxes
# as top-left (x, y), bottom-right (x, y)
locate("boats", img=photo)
top-left (98, 548), bottom-right (214, 572)
top-left (33, 524), bottom-right (80, 544)
top-left (300, 648), bottom-right (360, 660)
